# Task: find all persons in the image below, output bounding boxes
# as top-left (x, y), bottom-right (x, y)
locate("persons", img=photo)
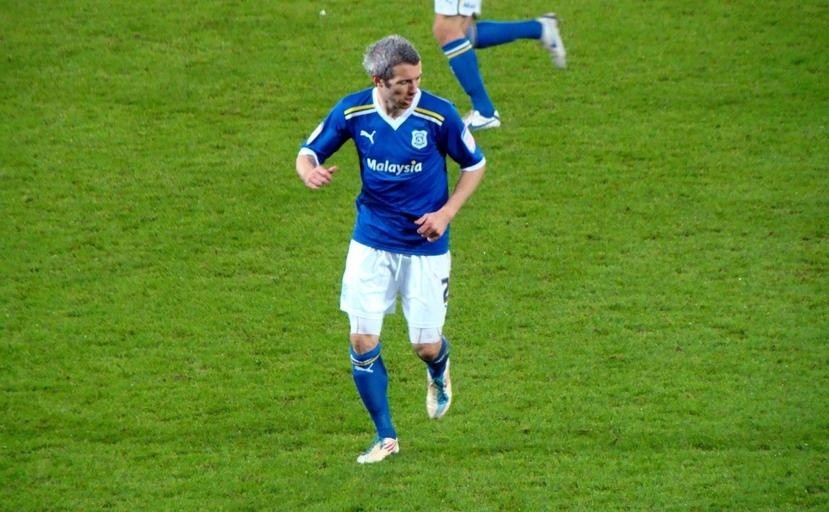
top-left (433, 1), bottom-right (567, 130)
top-left (295, 36), bottom-right (487, 465)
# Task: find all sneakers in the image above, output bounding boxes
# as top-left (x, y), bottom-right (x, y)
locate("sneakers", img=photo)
top-left (537, 13), bottom-right (566, 69)
top-left (357, 437), bottom-right (400, 464)
top-left (462, 109), bottom-right (500, 132)
top-left (426, 357), bottom-right (452, 419)
